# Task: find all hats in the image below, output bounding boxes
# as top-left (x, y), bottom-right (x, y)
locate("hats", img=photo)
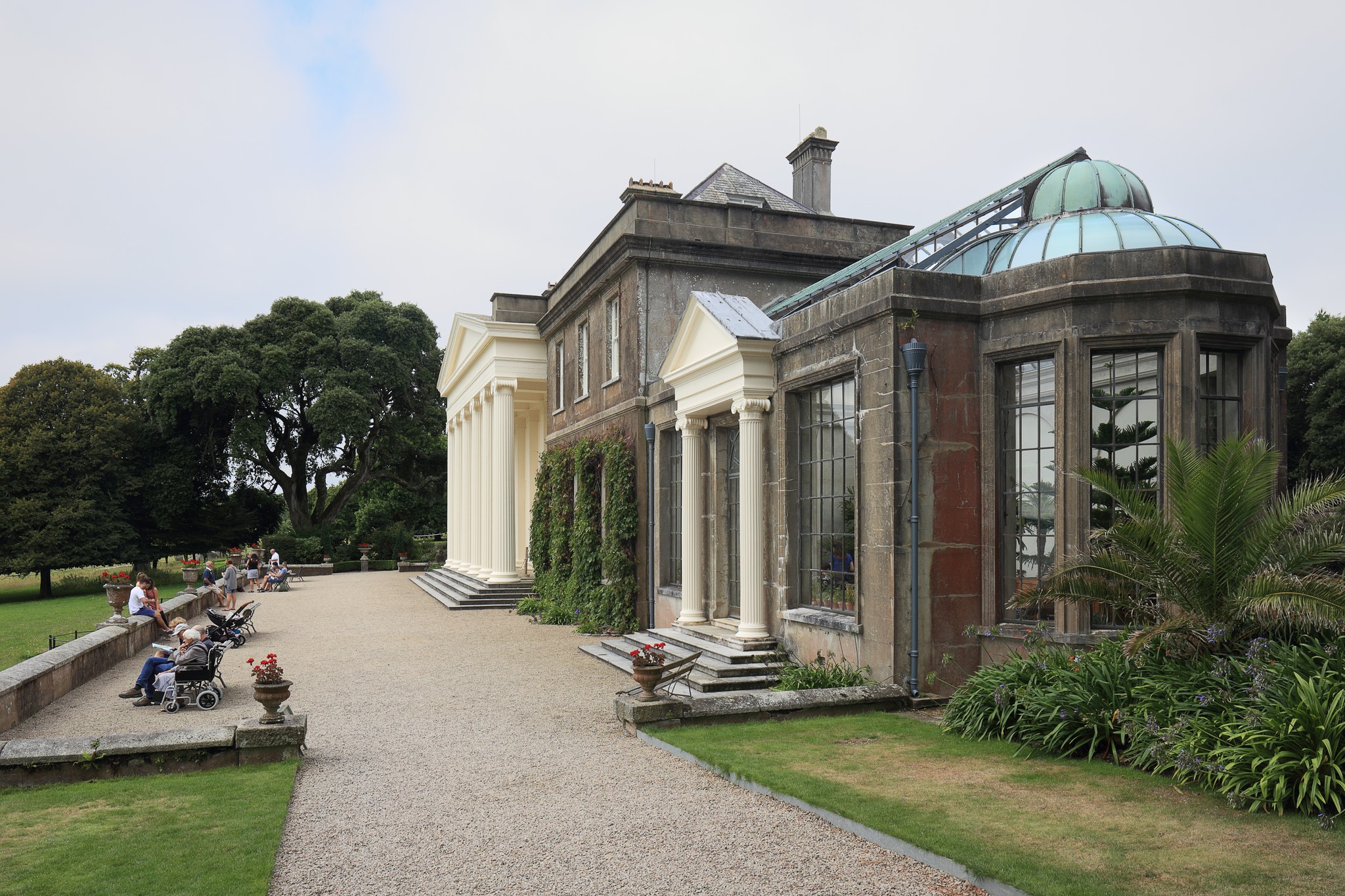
top-left (172, 623), bottom-right (189, 637)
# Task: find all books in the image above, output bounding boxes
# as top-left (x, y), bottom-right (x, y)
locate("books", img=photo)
top-left (152, 643), bottom-right (173, 653)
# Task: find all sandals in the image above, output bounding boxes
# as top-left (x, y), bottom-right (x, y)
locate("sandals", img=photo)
top-left (257, 587), bottom-right (264, 592)
top-left (160, 704), bottom-right (179, 712)
top-left (178, 700), bottom-right (186, 708)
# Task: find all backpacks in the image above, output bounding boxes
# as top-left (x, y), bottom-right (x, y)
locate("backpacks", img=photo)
top-left (279, 581), bottom-right (288, 592)
top-left (237, 585), bottom-right (244, 592)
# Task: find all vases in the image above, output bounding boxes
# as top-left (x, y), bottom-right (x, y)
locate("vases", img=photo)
top-left (323, 558), bottom-right (331, 563)
top-left (400, 556), bottom-right (407, 562)
top-left (812, 598), bottom-right (854, 611)
top-left (251, 680), bottom-right (293, 725)
top-left (103, 583), bottom-right (134, 623)
top-left (359, 548), bottom-right (370, 560)
top-left (181, 568), bottom-right (200, 592)
top-left (230, 554), bottom-right (243, 572)
top-left (630, 664), bottom-right (665, 702)
top-left (251, 548), bottom-right (265, 558)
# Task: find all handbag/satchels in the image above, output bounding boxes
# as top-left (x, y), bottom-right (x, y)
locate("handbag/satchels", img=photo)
top-left (266, 563), bottom-right (272, 574)
top-left (245, 566), bottom-right (248, 570)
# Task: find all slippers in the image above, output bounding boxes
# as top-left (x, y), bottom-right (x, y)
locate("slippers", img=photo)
top-left (222, 609), bottom-right (230, 611)
top-left (159, 633), bottom-right (163, 636)
top-left (229, 607), bottom-right (236, 610)
top-left (164, 629), bottom-right (175, 635)
top-left (248, 590), bottom-right (254, 592)
top-left (267, 590), bottom-right (272, 592)
top-left (218, 605), bottom-right (227, 607)
top-left (163, 637), bottom-right (171, 641)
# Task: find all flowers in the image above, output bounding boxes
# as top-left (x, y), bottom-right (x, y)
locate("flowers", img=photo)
top-left (252, 543), bottom-right (261, 548)
top-left (358, 543), bottom-right (370, 548)
top-left (230, 547), bottom-right (241, 554)
top-left (398, 552), bottom-right (408, 557)
top-left (811, 575), bottom-right (855, 604)
top-left (101, 572), bottom-right (131, 584)
top-left (630, 642), bottom-right (666, 667)
top-left (181, 560), bottom-right (200, 569)
top-left (324, 555), bottom-right (330, 558)
top-left (247, 653), bottom-right (284, 684)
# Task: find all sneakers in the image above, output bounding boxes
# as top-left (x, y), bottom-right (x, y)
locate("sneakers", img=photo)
top-left (119, 688), bottom-right (143, 698)
top-left (132, 695), bottom-right (153, 706)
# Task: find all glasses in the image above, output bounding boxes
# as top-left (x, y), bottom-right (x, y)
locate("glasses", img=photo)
top-left (211, 563), bottom-right (214, 564)
top-left (249, 555), bottom-right (251, 556)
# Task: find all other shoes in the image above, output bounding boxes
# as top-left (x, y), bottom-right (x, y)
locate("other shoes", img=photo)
top-left (264, 588), bottom-right (267, 591)
top-left (259, 582), bottom-right (262, 584)
top-left (254, 582), bottom-right (257, 584)
top-left (268, 589), bottom-right (270, 590)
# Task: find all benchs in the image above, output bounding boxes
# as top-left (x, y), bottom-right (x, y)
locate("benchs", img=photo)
top-left (235, 602), bottom-right (261, 641)
top-left (272, 565), bottom-right (305, 591)
top-left (183, 640), bottom-right (234, 688)
top-left (615, 650), bottom-right (703, 698)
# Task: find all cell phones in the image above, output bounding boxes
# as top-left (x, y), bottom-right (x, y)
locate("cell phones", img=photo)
top-left (221, 572), bottom-right (224, 573)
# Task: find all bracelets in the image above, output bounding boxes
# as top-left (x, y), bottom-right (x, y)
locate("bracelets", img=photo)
top-left (274, 573), bottom-right (275, 576)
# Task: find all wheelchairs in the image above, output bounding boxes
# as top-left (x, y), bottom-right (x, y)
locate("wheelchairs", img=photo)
top-left (151, 644), bottom-right (227, 713)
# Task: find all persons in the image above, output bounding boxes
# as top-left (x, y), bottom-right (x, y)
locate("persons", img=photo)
top-left (154, 616), bottom-right (191, 658)
top-left (268, 548), bottom-right (279, 571)
top-left (263, 562), bottom-right (283, 592)
top-left (245, 552), bottom-right (262, 584)
top-left (203, 560), bottom-right (228, 607)
top-left (245, 553), bottom-right (260, 592)
top-left (119, 625), bottom-right (215, 706)
top-left (257, 561), bottom-right (288, 592)
top-left (829, 541), bottom-right (854, 590)
top-left (222, 558), bottom-right (237, 611)
top-left (136, 572), bottom-right (171, 640)
top-left (154, 629), bottom-right (208, 712)
top-left (819, 562), bottom-right (836, 593)
top-left (128, 578), bottom-right (175, 634)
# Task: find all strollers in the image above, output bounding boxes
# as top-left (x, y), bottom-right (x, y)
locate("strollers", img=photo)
top-left (204, 600), bottom-right (254, 648)
